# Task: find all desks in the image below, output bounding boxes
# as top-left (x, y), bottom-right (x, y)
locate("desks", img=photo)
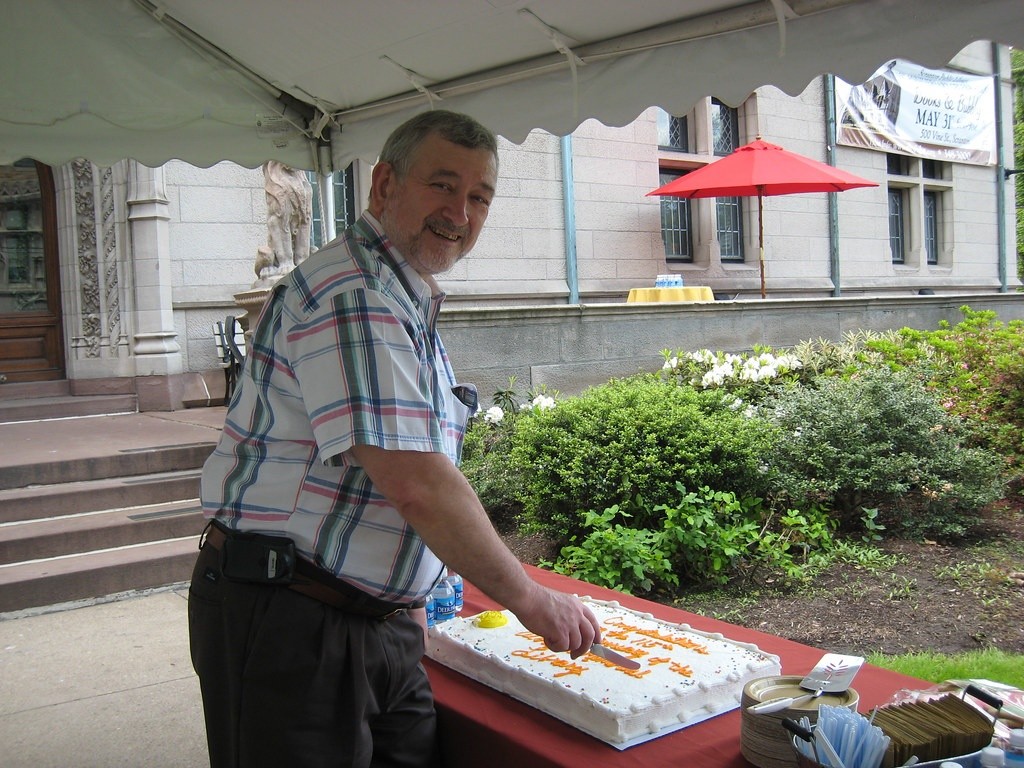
top-left (422, 561), bottom-right (939, 768)
top-left (626, 286), bottom-right (715, 302)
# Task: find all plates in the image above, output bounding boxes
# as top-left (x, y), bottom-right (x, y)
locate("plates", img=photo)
top-left (738, 675), bottom-right (860, 768)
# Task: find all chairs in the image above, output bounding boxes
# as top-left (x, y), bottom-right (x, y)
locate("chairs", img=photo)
top-left (212, 320), bottom-right (247, 407)
top-left (224, 316), bottom-right (245, 396)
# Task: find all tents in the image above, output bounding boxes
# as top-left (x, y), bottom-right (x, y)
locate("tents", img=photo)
top-left (0, 3), bottom-right (1024, 174)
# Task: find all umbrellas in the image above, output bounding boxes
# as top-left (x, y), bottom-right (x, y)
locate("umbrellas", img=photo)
top-left (645, 142), bottom-right (879, 299)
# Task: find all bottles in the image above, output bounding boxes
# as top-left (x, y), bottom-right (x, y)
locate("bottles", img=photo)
top-left (654, 274), bottom-right (684, 287)
top-left (1004, 729), bottom-right (1024, 768)
top-left (424, 591), bottom-right (434, 629)
top-left (940, 762), bottom-right (963, 768)
top-left (434, 577), bottom-right (455, 621)
top-left (445, 570), bottom-right (464, 614)
top-left (979, 747), bottom-right (1005, 768)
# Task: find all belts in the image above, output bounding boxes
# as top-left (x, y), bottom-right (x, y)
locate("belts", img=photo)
top-left (206, 526), bottom-right (406, 621)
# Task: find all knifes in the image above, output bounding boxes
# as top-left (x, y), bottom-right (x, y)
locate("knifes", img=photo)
top-left (589, 643), bottom-right (640, 670)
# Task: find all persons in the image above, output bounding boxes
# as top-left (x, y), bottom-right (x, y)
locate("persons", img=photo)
top-left (191, 111), bottom-right (602, 767)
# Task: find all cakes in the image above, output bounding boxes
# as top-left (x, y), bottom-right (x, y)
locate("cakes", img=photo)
top-left (421, 594), bottom-right (782, 743)
